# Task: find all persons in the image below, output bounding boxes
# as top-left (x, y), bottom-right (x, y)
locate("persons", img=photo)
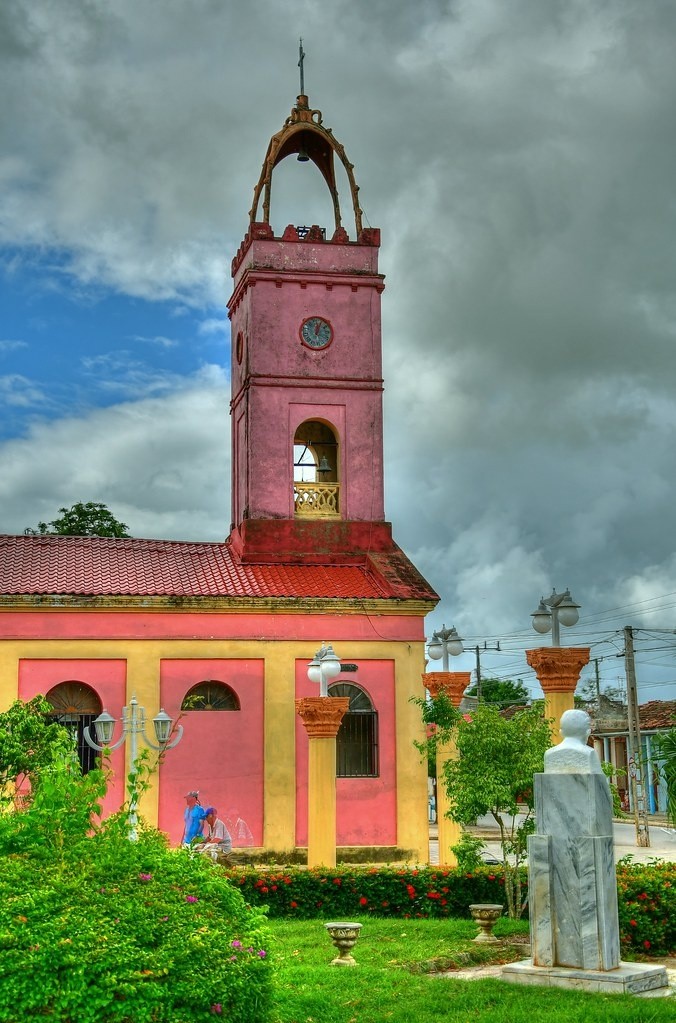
top-left (544, 709), bottom-right (604, 774)
top-left (180, 790), bottom-right (205, 848)
top-left (200, 807), bottom-right (232, 855)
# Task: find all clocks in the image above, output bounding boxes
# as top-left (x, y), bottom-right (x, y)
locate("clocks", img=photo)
top-left (300, 316), bottom-right (335, 350)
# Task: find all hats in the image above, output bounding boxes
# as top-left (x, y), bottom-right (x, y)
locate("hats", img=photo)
top-left (202, 808), bottom-right (217, 817)
top-left (184, 791), bottom-right (198, 799)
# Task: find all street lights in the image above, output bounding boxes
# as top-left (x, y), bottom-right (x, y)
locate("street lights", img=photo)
top-left (531, 586), bottom-right (582, 649)
top-left (308, 646), bottom-right (342, 697)
top-left (83, 697), bottom-right (184, 834)
top-left (427, 623), bottom-right (463, 672)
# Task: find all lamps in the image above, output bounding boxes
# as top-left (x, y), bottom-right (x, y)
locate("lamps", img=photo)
top-left (529, 586), bottom-right (582, 647)
top-left (425, 623), bottom-right (464, 672)
top-left (305, 641), bottom-right (342, 698)
